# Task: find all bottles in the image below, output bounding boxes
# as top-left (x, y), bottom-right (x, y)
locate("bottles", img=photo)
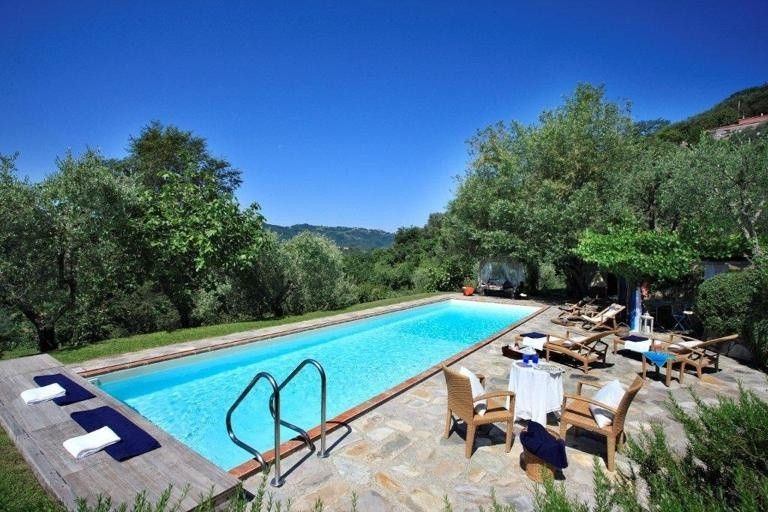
top-left (523, 353), bottom-right (529, 368)
top-left (532, 354), bottom-right (540, 368)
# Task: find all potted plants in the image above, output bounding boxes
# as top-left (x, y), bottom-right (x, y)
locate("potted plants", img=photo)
top-left (462, 278), bottom-right (476, 296)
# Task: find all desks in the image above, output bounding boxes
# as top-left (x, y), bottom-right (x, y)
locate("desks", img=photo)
top-left (515, 360), bottom-right (564, 424)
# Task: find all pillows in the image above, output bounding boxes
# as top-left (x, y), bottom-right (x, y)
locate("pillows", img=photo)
top-left (589, 379), bottom-right (626, 428)
top-left (459, 366), bottom-right (488, 417)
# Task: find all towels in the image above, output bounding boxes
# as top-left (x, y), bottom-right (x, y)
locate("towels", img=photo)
top-left (19, 373), bottom-right (161, 463)
top-left (520, 420), bottom-right (568, 468)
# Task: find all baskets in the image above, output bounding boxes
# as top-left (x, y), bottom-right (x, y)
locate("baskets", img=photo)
top-left (519, 426), bottom-right (562, 483)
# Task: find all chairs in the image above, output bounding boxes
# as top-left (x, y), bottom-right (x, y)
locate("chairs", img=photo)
top-left (561, 374), bottom-right (645, 472)
top-left (501, 296), bottom-right (738, 389)
top-left (440, 364), bottom-right (517, 459)
